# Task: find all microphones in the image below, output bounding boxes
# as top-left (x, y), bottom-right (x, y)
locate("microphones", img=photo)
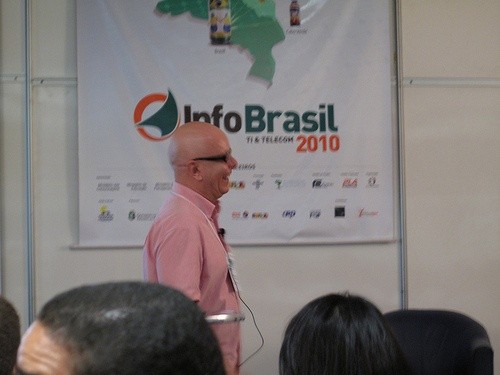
top-left (219, 228), bottom-right (225, 235)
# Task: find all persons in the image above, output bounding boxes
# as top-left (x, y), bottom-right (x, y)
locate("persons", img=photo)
top-left (279, 294), bottom-right (407, 375)
top-left (210, 11), bottom-right (231, 32)
top-left (143, 121), bottom-right (241, 375)
top-left (13, 282), bottom-right (226, 375)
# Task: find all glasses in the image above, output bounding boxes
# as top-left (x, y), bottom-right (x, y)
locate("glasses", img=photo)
top-left (191, 149), bottom-right (232, 163)
